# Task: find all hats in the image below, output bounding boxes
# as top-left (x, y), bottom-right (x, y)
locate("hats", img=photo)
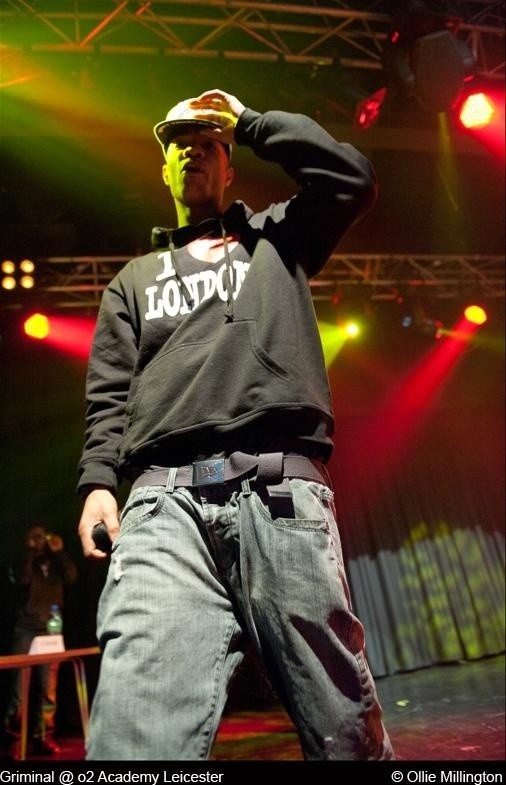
top-left (153, 95), bottom-right (233, 160)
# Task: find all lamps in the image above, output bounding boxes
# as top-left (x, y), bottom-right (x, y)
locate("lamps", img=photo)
top-left (396, 288), bottom-right (445, 341)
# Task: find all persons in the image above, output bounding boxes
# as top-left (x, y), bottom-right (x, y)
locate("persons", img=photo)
top-left (70, 86), bottom-right (386, 762)
top-left (0, 523), bottom-right (75, 760)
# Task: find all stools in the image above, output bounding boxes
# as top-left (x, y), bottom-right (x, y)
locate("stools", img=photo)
top-left (1, 645), bottom-right (102, 761)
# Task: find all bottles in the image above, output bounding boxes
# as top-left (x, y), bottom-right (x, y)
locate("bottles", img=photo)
top-left (47, 605), bottom-right (63, 635)
